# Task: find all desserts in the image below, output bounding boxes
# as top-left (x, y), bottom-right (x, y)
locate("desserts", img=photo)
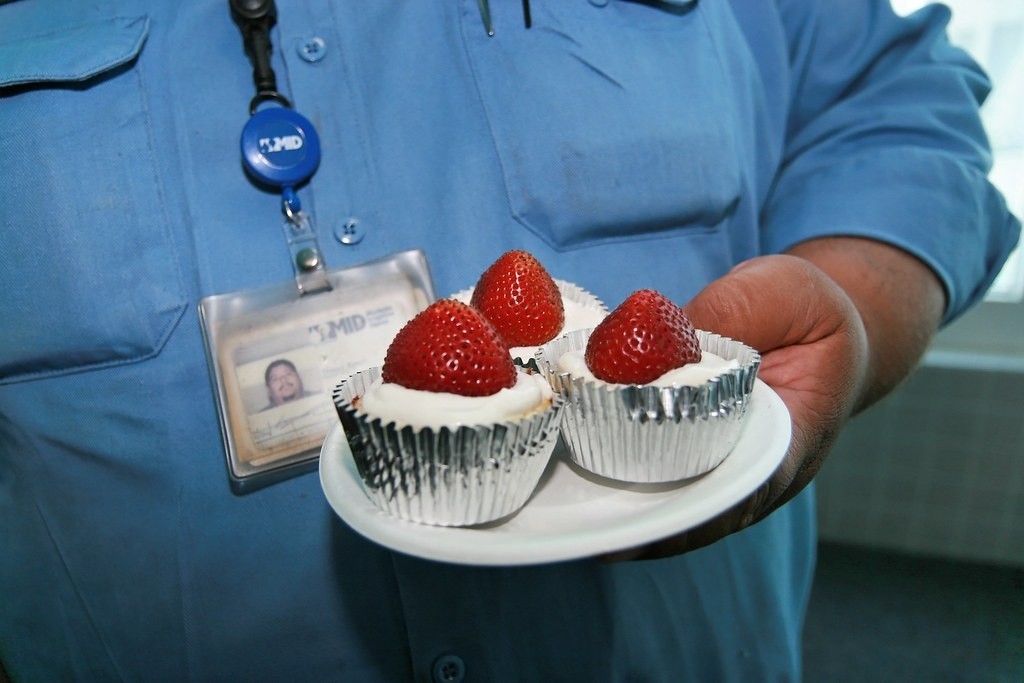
top-left (535, 289), bottom-right (761, 484)
top-left (445, 249), bottom-right (610, 372)
top-left (330, 298), bottom-right (564, 525)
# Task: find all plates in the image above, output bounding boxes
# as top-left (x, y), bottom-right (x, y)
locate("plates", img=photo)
top-left (313, 375), bottom-right (793, 568)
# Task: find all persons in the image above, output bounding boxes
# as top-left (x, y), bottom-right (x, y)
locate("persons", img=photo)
top-left (262, 361), bottom-right (316, 411)
top-left (0, 0), bottom-right (1022, 682)
